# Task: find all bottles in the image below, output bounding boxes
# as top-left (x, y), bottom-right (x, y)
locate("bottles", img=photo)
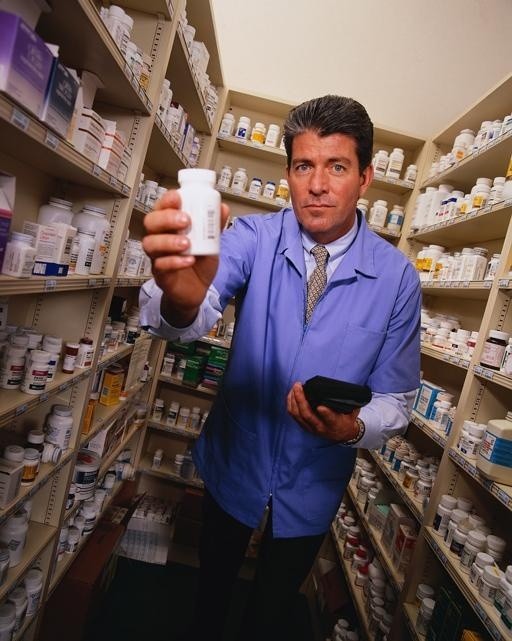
top-left (217, 109), bottom-right (418, 234)
top-left (101, 5), bottom-right (221, 168)
top-left (331, 392), bottom-right (512, 640)
top-left (210, 318), bottom-right (235, 342)
top-left (175, 168), bottom-right (221, 255)
top-left (151, 398), bottom-right (210, 476)
top-left (2, 173), bottom-right (167, 280)
top-left (0, 405), bottom-right (135, 641)
top-left (413, 243), bottom-right (512, 378)
top-left (410, 112), bottom-right (512, 231)
top-left (0, 304), bottom-right (145, 395)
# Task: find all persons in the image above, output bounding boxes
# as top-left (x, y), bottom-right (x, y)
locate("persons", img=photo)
top-left (138, 95), bottom-right (423, 641)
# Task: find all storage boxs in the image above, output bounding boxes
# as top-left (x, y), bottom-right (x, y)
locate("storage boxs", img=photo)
top-left (114, 494), bottom-right (181, 567)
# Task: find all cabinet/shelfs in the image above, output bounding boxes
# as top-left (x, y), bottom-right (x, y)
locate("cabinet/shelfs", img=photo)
top-left (109, 89), bottom-right (429, 593)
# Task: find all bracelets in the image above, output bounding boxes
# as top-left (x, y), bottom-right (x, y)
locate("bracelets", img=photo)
top-left (341, 419), bottom-right (365, 446)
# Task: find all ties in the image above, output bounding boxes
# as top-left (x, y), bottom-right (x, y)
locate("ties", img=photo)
top-left (305, 246), bottom-right (328, 323)
